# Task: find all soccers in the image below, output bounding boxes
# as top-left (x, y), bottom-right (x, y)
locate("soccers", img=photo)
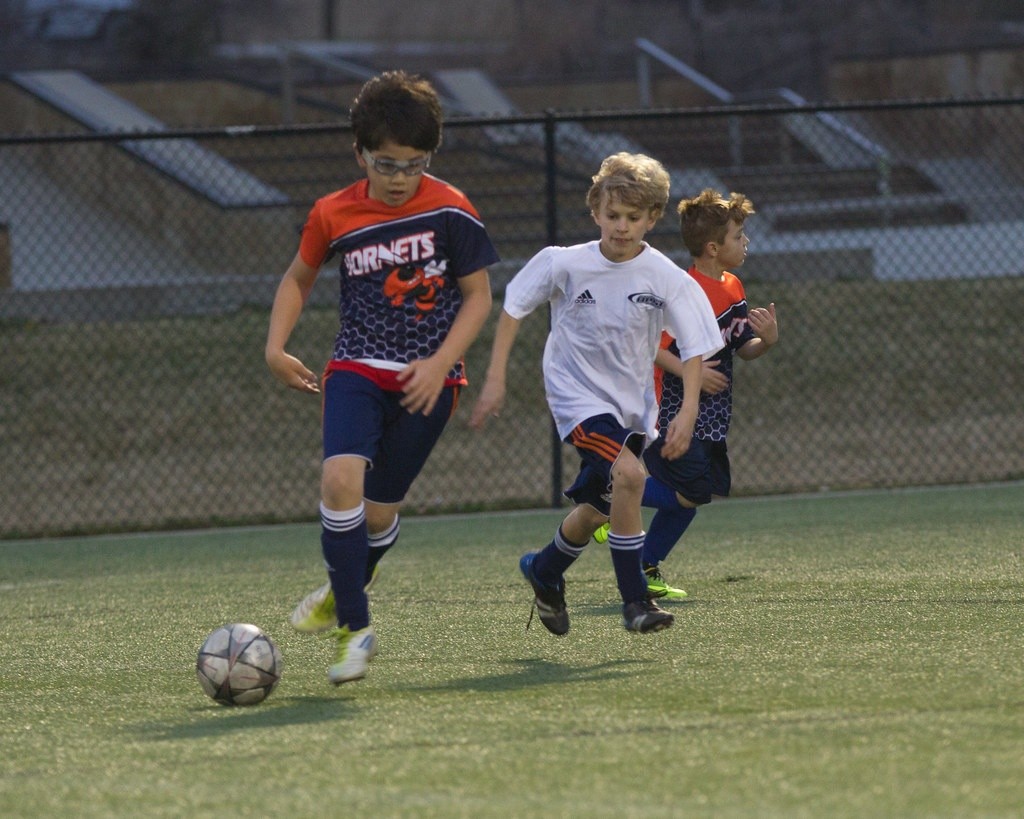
top-left (195, 620), bottom-right (284, 708)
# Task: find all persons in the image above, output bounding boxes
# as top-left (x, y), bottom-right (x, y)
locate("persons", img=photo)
top-left (593, 186), bottom-right (779, 601)
top-left (468, 151), bottom-right (726, 635)
top-left (263, 68), bottom-right (502, 685)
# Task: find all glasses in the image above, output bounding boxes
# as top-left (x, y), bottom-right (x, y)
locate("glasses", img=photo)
top-left (360, 148), bottom-right (432, 176)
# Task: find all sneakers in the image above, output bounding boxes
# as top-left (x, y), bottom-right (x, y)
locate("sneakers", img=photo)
top-left (593, 522), bottom-right (611, 543)
top-left (519, 552), bottom-right (569, 635)
top-left (622, 599), bottom-right (675, 634)
top-left (328, 624), bottom-right (378, 682)
top-left (645, 567), bottom-right (687, 599)
top-left (291, 562), bottom-right (378, 631)
